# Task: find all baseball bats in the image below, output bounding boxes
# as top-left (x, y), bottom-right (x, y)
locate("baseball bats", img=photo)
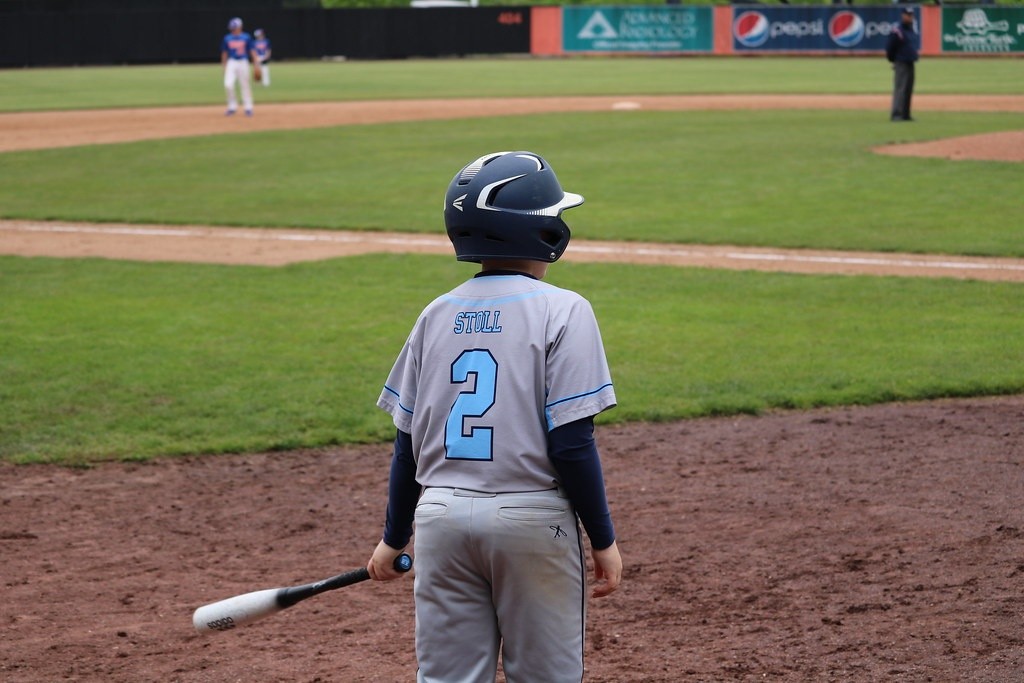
top-left (192, 553), bottom-right (412, 635)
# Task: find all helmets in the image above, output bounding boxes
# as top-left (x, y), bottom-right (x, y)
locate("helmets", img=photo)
top-left (444, 151), bottom-right (584, 264)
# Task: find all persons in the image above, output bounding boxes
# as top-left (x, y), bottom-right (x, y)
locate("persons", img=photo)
top-left (221, 17), bottom-right (273, 118)
top-left (366, 148), bottom-right (622, 683)
top-left (884, 6), bottom-right (921, 123)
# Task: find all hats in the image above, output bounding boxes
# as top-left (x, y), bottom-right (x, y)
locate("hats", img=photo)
top-left (228, 18), bottom-right (243, 30)
top-left (902, 7), bottom-right (915, 15)
top-left (254, 29), bottom-right (264, 37)
